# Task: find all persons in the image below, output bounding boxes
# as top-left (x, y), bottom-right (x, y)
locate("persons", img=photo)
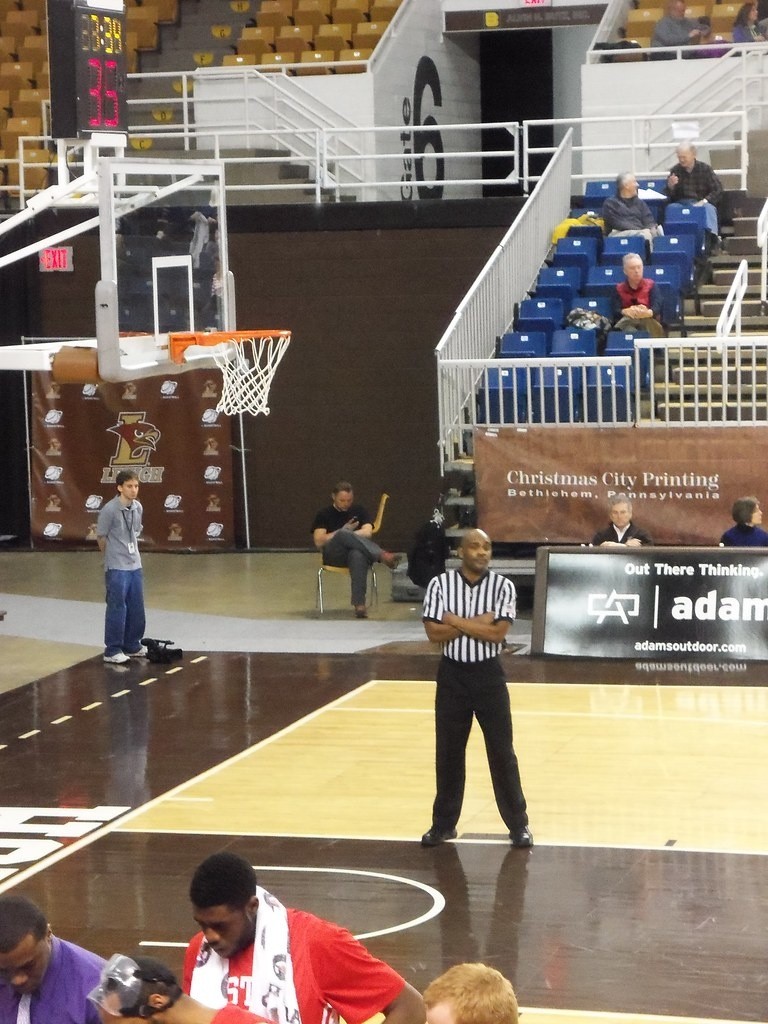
top-left (0, 893), bottom-right (110, 1024)
top-left (84, 954), bottom-right (278, 1024)
top-left (416, 526), bottom-right (533, 848)
top-left (661, 140), bottom-right (725, 235)
top-left (718, 496), bottom-right (768, 546)
top-left (599, 173), bottom-right (659, 252)
top-left (650, 0), bottom-right (711, 59)
top-left (180, 851), bottom-right (427, 1024)
top-left (307, 481), bottom-right (403, 618)
top-left (422, 962), bottom-right (519, 1024)
top-left (755, 0), bottom-right (768, 35)
top-left (592, 494), bottom-right (654, 547)
top-left (733, 1), bottom-right (765, 42)
top-left (612, 251), bottom-right (667, 339)
top-left (96, 469), bottom-right (150, 664)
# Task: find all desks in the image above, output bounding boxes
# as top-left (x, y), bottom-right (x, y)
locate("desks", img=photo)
top-left (530, 545), bottom-right (768, 663)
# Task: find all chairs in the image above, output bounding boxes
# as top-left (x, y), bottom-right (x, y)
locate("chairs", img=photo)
top-left (316, 494), bottom-right (390, 614)
top-left (0, 0), bottom-right (176, 198)
top-left (614, 1), bottom-right (755, 60)
top-left (474, 181), bottom-right (716, 423)
top-left (116, 217), bottom-right (222, 332)
top-left (222, 0), bottom-right (403, 76)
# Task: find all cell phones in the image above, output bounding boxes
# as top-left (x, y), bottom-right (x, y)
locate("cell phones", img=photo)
top-left (352, 516), bottom-right (358, 523)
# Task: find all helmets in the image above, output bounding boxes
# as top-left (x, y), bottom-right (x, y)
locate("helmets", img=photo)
top-left (103, 955), bottom-right (182, 1017)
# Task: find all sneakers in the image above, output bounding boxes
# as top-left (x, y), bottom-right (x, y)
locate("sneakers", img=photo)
top-left (421, 827), bottom-right (458, 847)
top-left (510, 826), bottom-right (533, 847)
top-left (355, 602), bottom-right (368, 618)
top-left (103, 651), bottom-right (131, 663)
top-left (380, 550), bottom-right (400, 569)
top-left (124, 646), bottom-right (147, 658)
